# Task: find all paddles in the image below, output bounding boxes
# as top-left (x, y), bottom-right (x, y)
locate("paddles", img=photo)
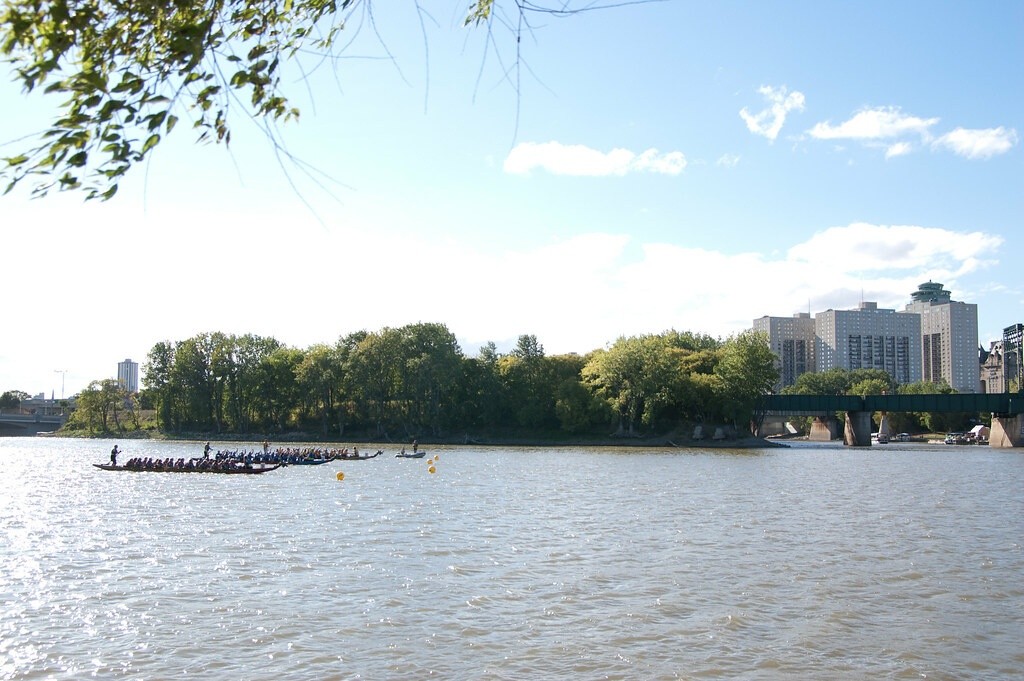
top-left (203, 450), bottom-right (211, 458)
top-left (106, 451), bottom-right (121, 465)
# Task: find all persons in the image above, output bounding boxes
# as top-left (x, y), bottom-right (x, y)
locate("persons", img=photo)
top-left (111, 445), bottom-right (119, 466)
top-left (401, 447), bottom-right (405, 455)
top-left (970, 435), bottom-right (980, 443)
top-left (126, 445), bottom-right (348, 469)
top-left (350, 447), bottom-right (359, 457)
top-left (204, 442), bottom-right (213, 460)
top-left (413, 440), bottom-right (418, 453)
top-left (264, 439), bottom-right (270, 453)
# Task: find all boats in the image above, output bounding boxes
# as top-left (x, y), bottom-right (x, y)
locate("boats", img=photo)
top-left (396, 452), bottom-right (426, 459)
top-left (90, 462), bottom-right (288, 474)
top-left (877, 433), bottom-right (888, 444)
top-left (190, 455), bottom-right (339, 465)
top-left (945, 432), bottom-right (989, 445)
top-left (336, 451), bottom-right (384, 460)
